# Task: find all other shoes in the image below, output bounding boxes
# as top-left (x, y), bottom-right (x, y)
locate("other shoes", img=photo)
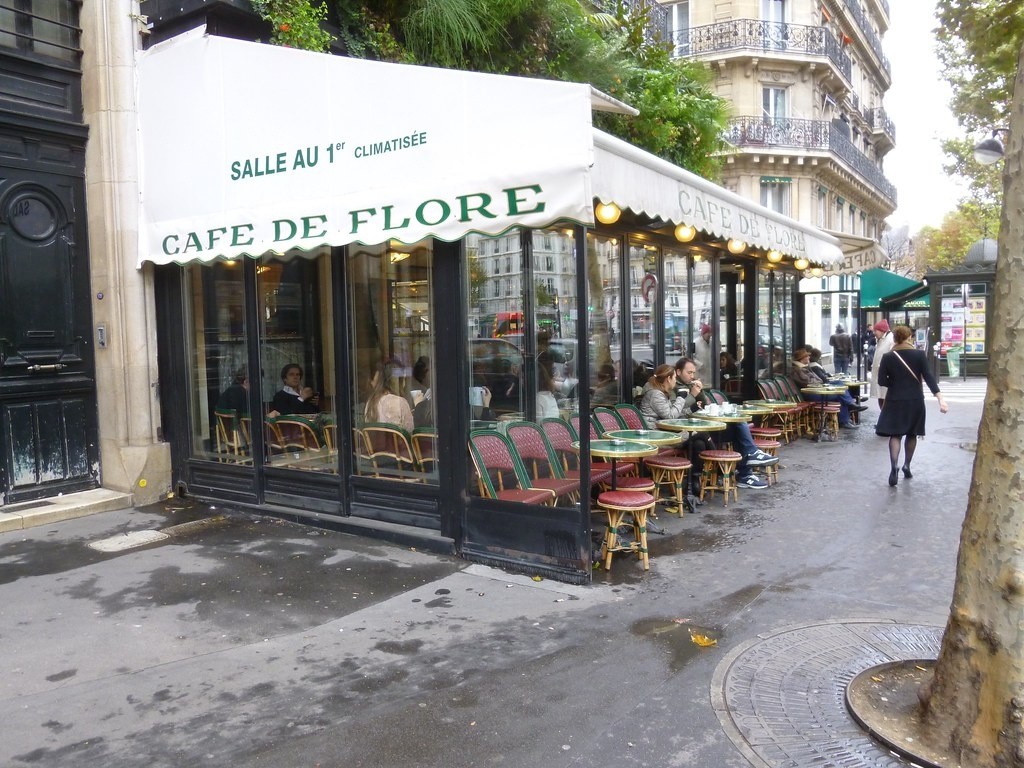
top-left (860, 396), bottom-right (868, 402)
top-left (685, 474), bottom-right (703, 496)
top-left (849, 404), bottom-right (868, 413)
top-left (839, 422), bottom-right (860, 429)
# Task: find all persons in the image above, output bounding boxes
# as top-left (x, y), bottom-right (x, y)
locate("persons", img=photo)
top-left (413, 386), bottom-right (492, 459)
top-left (808, 348), bottom-right (869, 402)
top-left (536, 362), bottom-right (560, 425)
top-left (535, 332), bottom-right (566, 380)
top-left (615, 358), bottom-right (650, 405)
top-left (672, 358), bottom-right (780, 489)
top-left (720, 352), bottom-right (738, 392)
top-left (638, 365), bottom-right (740, 494)
top-left (791, 349), bottom-right (868, 429)
top-left (273, 363), bottom-right (319, 415)
top-left (870, 319), bottom-right (896, 427)
top-left (829, 323), bottom-right (854, 373)
top-left (693, 324), bottom-right (712, 385)
top-left (852, 324), bottom-right (876, 372)
top-left (875, 326), bottom-right (949, 486)
top-left (364, 360), bottom-right (414, 459)
top-left (218, 367), bottom-right (281, 463)
top-left (590, 365), bottom-right (618, 411)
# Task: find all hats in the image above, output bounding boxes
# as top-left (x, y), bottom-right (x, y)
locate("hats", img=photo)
top-left (874, 319), bottom-right (889, 332)
top-left (701, 325), bottom-right (710, 334)
top-left (794, 349), bottom-right (811, 360)
top-left (836, 323), bottom-right (844, 330)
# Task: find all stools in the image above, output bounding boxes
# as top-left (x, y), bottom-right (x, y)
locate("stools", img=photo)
top-left (600, 403), bottom-right (841, 570)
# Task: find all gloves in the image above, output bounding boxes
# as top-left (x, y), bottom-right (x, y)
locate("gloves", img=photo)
top-left (850, 358), bottom-right (853, 363)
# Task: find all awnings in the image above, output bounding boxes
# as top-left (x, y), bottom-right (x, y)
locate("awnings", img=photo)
top-left (860, 267), bottom-right (924, 309)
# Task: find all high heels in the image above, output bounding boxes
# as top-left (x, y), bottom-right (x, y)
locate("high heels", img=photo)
top-left (902, 465), bottom-right (912, 479)
top-left (889, 466), bottom-right (899, 486)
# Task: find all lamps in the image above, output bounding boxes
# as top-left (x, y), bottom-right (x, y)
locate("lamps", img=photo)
top-left (595, 202), bottom-right (826, 277)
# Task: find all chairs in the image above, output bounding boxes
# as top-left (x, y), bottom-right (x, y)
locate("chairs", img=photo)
top-left (208, 379), bottom-right (816, 507)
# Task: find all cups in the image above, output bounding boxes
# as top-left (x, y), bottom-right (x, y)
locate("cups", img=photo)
top-left (701, 402), bottom-right (738, 418)
top-left (410, 390), bottom-right (422, 401)
top-left (679, 388), bottom-right (690, 395)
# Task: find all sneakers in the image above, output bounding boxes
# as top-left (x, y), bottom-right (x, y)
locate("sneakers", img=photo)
top-left (746, 450), bottom-right (779, 467)
top-left (737, 474), bottom-right (767, 489)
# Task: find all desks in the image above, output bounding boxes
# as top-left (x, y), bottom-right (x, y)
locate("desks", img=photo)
top-left (572, 377), bottom-right (875, 531)
